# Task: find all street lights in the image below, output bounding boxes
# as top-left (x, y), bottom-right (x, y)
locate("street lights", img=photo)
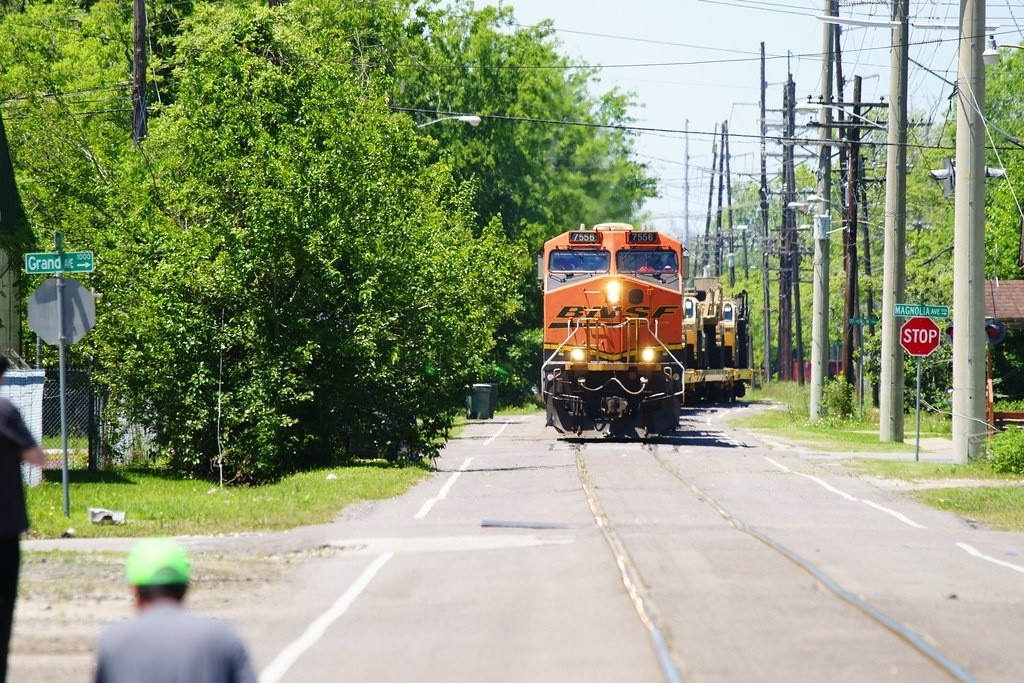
top-left (808, 193), bottom-right (869, 422)
top-left (794, 103), bottom-right (906, 443)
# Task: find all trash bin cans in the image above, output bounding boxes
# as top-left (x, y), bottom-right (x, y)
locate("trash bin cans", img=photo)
top-left (792, 360), bottom-right (842, 382)
top-left (471, 383), bottom-right (498, 420)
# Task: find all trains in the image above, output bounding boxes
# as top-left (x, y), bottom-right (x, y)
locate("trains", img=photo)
top-left (538, 221), bottom-right (687, 441)
top-left (683, 263), bottom-right (757, 405)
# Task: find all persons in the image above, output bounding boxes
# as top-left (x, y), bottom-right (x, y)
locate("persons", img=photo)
top-left (89, 534), bottom-right (263, 683)
top-left (0, 355), bottom-right (46, 683)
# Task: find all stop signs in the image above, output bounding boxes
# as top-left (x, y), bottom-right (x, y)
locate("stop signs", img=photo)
top-left (899, 315), bottom-right (942, 358)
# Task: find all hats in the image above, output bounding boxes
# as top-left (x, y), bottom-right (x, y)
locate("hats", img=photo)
top-left (124, 537), bottom-right (190, 586)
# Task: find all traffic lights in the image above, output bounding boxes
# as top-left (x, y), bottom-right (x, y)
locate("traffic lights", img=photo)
top-left (929, 155), bottom-right (956, 196)
top-left (946, 320), bottom-right (955, 345)
top-left (986, 319), bottom-right (1007, 347)
top-left (985, 164), bottom-right (1007, 182)
